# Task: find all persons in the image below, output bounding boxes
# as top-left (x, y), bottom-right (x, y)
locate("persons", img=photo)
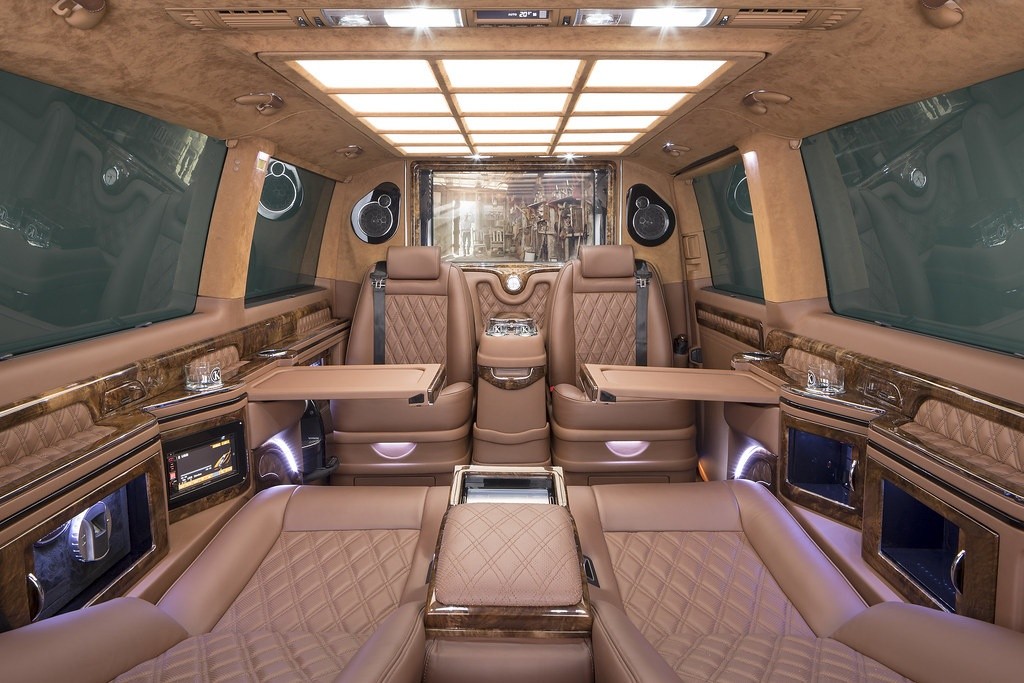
top-left (460, 213), bottom-right (472, 256)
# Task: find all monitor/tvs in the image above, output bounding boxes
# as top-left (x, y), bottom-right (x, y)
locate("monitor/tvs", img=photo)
top-left (162, 420), bottom-right (248, 511)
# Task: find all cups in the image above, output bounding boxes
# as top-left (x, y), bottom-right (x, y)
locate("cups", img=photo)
top-left (806, 363), bottom-right (846, 395)
top-left (183, 360), bottom-right (221, 387)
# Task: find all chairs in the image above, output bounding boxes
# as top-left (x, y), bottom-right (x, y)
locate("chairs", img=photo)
top-left (547, 243), bottom-right (693, 485)
top-left (0, 484), bottom-right (451, 683)
top-left (330, 246), bottom-right (475, 485)
top-left (566, 478), bottom-right (1024, 683)
